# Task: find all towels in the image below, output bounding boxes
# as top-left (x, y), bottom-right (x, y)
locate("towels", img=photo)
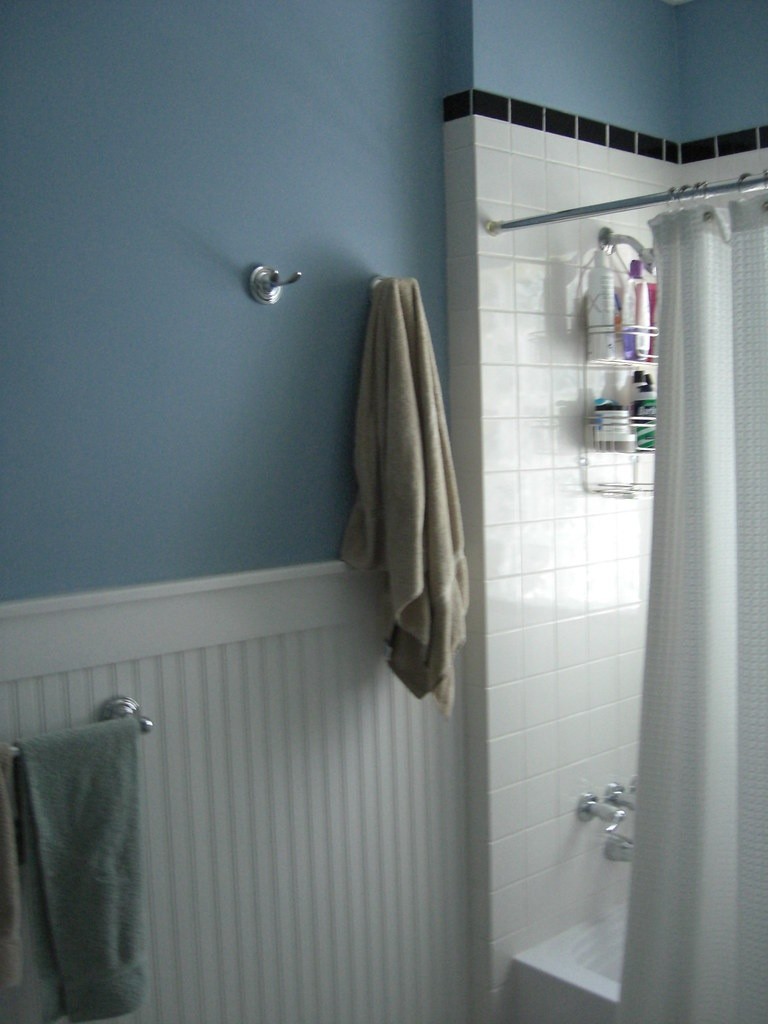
top-left (1, 709), bottom-right (154, 1023)
top-left (334, 273), bottom-right (473, 718)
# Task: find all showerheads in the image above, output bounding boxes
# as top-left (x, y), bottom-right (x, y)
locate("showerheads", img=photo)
top-left (598, 226), bottom-right (658, 276)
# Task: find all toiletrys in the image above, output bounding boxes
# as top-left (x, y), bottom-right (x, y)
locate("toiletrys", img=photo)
top-left (594, 368), bottom-right (657, 454)
top-left (584, 245), bottom-right (654, 368)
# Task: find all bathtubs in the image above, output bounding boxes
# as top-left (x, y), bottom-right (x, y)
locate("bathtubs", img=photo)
top-left (512, 896), bottom-right (629, 1023)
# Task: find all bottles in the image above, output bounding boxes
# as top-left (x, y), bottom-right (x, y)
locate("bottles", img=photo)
top-left (621, 260), bottom-right (650, 361)
top-left (635, 385), bottom-right (656, 447)
top-left (629, 371), bottom-right (647, 422)
top-left (588, 252), bottom-right (614, 360)
